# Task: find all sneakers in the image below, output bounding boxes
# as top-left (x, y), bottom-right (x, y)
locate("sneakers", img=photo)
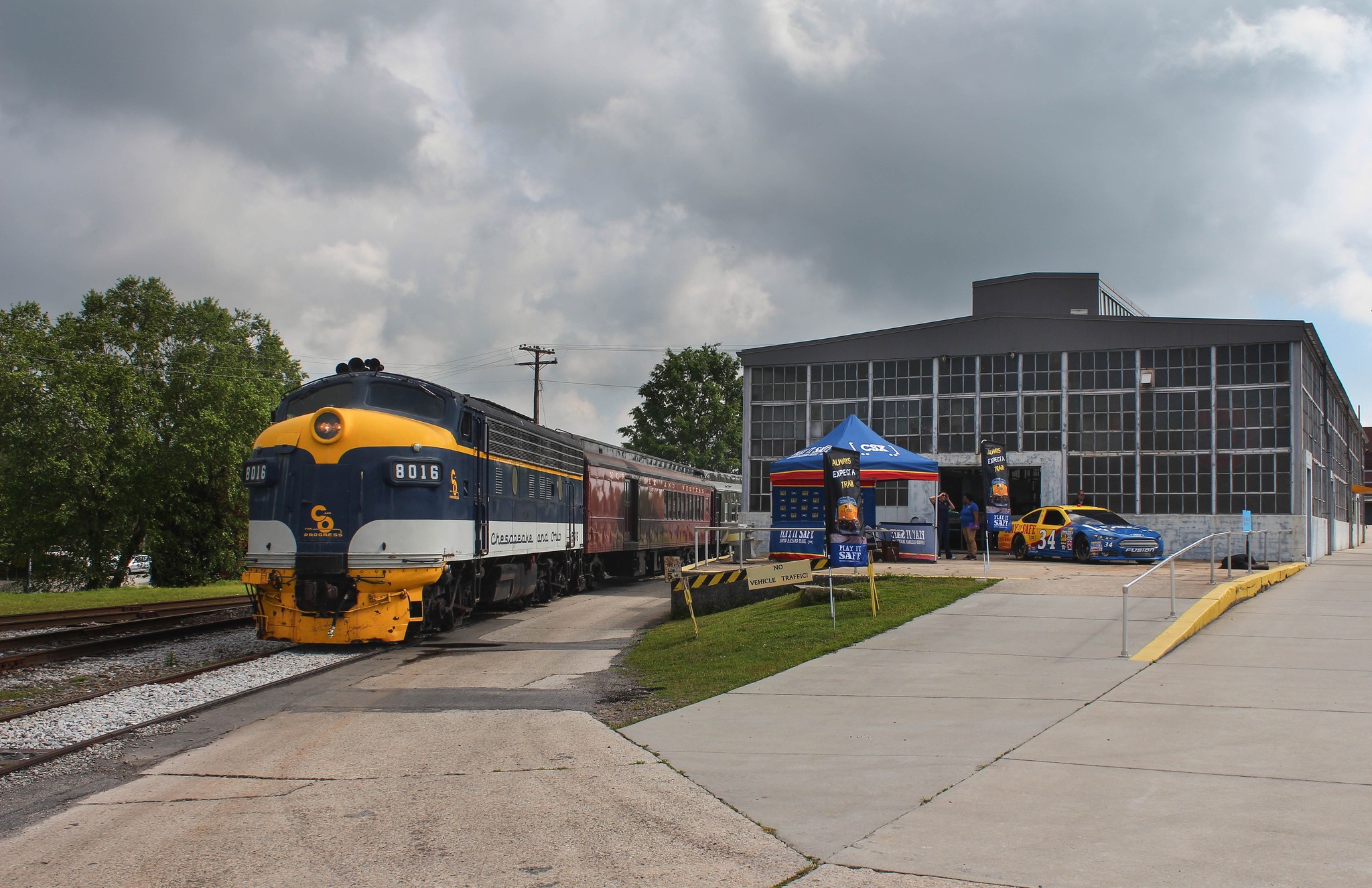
top-left (962, 554), bottom-right (972, 560)
top-left (936, 556), bottom-right (941, 560)
top-left (968, 555), bottom-right (978, 560)
top-left (946, 556), bottom-right (956, 560)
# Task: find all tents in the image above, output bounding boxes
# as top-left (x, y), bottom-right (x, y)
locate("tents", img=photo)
top-left (768, 414), bottom-right (940, 564)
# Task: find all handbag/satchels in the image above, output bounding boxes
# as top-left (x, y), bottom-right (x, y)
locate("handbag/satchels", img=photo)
top-left (969, 521), bottom-right (980, 530)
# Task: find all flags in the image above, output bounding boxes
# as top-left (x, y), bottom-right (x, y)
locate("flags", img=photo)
top-left (981, 440), bottom-right (1013, 532)
top-left (822, 446), bottom-right (870, 568)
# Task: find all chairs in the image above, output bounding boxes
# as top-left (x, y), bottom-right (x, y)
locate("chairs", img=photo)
top-left (865, 525), bottom-right (878, 551)
top-left (947, 511), bottom-right (964, 549)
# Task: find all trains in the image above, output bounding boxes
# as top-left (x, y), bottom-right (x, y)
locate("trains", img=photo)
top-left (990, 478), bottom-right (1008, 508)
top-left (241, 355), bottom-right (744, 650)
top-left (835, 497), bottom-right (861, 534)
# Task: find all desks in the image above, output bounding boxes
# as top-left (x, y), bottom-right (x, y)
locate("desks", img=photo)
top-left (865, 530), bottom-right (904, 563)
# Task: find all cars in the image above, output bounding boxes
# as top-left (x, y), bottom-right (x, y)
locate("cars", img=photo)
top-left (995, 504), bottom-right (1165, 564)
top-left (106, 554), bottom-right (153, 574)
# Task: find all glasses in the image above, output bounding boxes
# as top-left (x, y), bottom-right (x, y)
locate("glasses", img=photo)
top-left (1078, 493), bottom-right (1085, 496)
top-left (939, 494), bottom-right (947, 497)
top-left (963, 496), bottom-right (969, 499)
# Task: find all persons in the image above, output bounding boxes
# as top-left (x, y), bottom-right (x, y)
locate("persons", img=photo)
top-left (1071, 490), bottom-right (1091, 507)
top-left (928, 491), bottom-right (957, 560)
top-left (960, 493), bottom-right (980, 560)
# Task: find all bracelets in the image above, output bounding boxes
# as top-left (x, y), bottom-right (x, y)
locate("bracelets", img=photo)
top-left (974, 525), bottom-right (977, 527)
